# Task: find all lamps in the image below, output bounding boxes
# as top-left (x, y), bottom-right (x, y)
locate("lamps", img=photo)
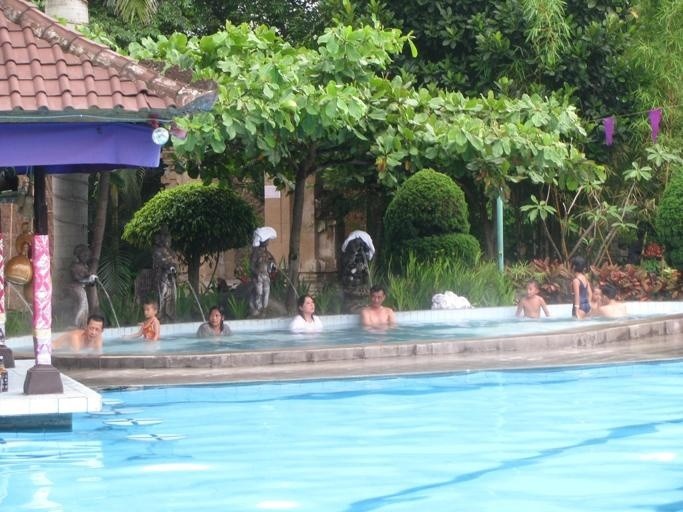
top-left (149, 115), bottom-right (187, 146)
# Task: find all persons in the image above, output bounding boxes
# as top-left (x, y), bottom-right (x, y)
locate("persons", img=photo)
top-left (118, 300), bottom-right (160, 342)
top-left (569, 256), bottom-right (592, 319)
top-left (358, 284), bottom-right (396, 325)
top-left (246, 229), bottom-right (277, 317)
top-left (289, 294), bottom-right (322, 333)
top-left (194, 305), bottom-right (231, 337)
top-left (63, 242), bottom-right (98, 327)
top-left (49, 315), bottom-right (104, 352)
top-left (340, 237), bottom-right (371, 313)
top-left (514, 278), bottom-right (551, 319)
top-left (590, 286), bottom-right (604, 315)
top-left (150, 230), bottom-right (180, 323)
